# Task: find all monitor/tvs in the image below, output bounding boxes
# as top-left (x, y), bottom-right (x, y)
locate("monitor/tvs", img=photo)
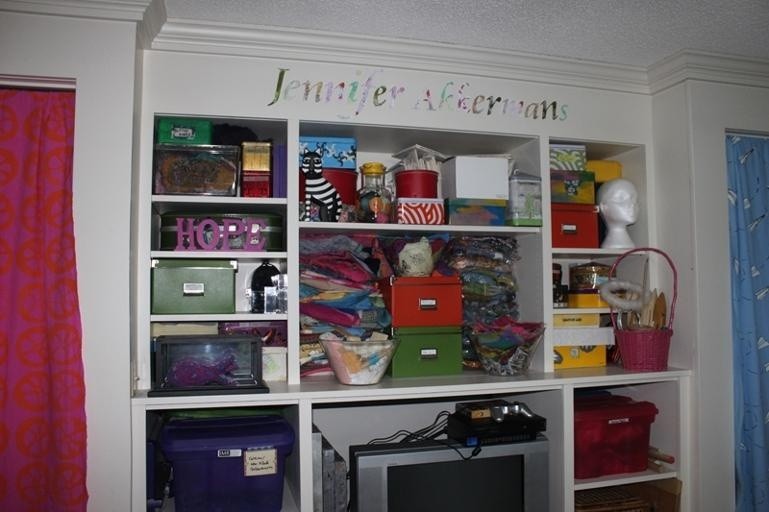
top-left (347, 434), bottom-right (552, 512)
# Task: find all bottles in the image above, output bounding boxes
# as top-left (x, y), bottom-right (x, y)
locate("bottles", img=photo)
top-left (355, 160), bottom-right (391, 223)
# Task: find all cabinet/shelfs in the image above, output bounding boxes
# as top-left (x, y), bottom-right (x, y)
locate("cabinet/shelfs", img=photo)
top-left (129, 1), bottom-right (693, 512)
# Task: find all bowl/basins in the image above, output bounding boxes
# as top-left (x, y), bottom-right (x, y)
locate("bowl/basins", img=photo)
top-left (317, 336), bottom-right (402, 385)
top-left (464, 326), bottom-right (546, 378)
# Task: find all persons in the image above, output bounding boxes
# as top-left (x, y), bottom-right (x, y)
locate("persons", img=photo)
top-left (596, 178), bottom-right (639, 248)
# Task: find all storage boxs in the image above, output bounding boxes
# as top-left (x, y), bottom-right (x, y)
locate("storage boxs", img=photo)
top-left (550, 169), bottom-right (595, 205)
top-left (299, 136), bottom-right (358, 169)
top-left (149, 258), bottom-right (238, 313)
top-left (443, 196), bottom-right (506, 226)
top-left (154, 116), bottom-right (210, 144)
top-left (439, 155), bottom-right (510, 200)
top-left (573, 389), bottom-right (658, 479)
top-left (551, 202), bottom-right (600, 248)
top-left (554, 327), bottom-right (614, 369)
top-left (158, 414), bottom-right (296, 512)
top-left (389, 197), bottom-right (445, 224)
top-left (385, 326), bottom-right (463, 377)
top-left (298, 167), bottom-right (359, 206)
top-left (549, 143), bottom-right (588, 171)
top-left (587, 158), bottom-right (622, 182)
top-left (381, 274), bottom-right (464, 327)
top-left (506, 174), bottom-right (543, 226)
top-left (152, 142), bottom-right (238, 196)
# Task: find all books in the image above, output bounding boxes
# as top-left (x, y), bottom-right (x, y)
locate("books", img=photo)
top-left (312, 423), bottom-right (347, 512)
